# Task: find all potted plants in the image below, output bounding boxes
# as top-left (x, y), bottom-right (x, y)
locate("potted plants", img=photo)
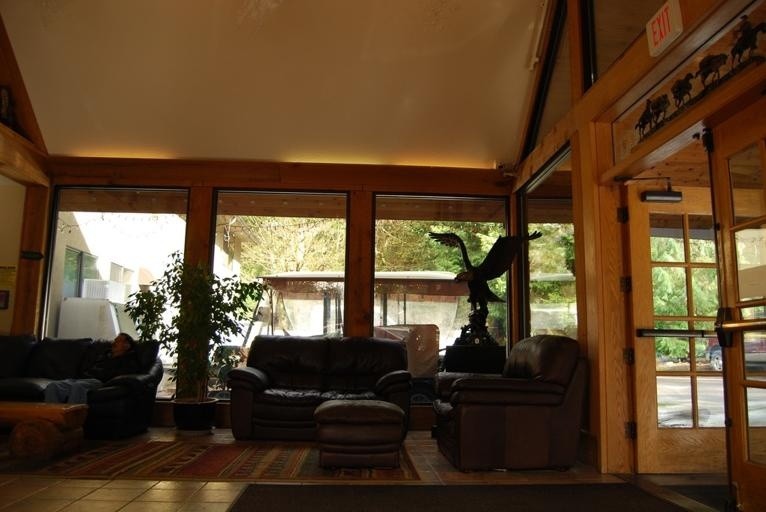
top-left (124, 253), bottom-right (270, 438)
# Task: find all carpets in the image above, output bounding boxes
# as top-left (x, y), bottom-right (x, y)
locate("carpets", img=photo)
top-left (661, 483), bottom-right (729, 512)
top-left (225, 482), bottom-right (693, 512)
top-left (0, 440), bottom-right (423, 481)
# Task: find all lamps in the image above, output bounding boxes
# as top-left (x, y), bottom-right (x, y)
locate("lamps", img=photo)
top-left (614, 174), bottom-right (682, 202)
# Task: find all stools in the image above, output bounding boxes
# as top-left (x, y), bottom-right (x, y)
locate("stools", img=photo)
top-left (313, 399), bottom-right (406, 472)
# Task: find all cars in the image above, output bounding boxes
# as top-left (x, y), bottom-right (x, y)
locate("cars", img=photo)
top-left (704, 317), bottom-right (766, 372)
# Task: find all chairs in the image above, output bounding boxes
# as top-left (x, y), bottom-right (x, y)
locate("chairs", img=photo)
top-left (433, 335), bottom-right (590, 473)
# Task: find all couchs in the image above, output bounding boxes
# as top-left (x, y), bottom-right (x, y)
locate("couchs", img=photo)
top-left (227, 334), bottom-right (412, 443)
top-left (0, 334), bottom-right (164, 442)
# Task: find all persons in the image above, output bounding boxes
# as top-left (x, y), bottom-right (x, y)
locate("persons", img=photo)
top-left (44, 332), bottom-right (160, 408)
